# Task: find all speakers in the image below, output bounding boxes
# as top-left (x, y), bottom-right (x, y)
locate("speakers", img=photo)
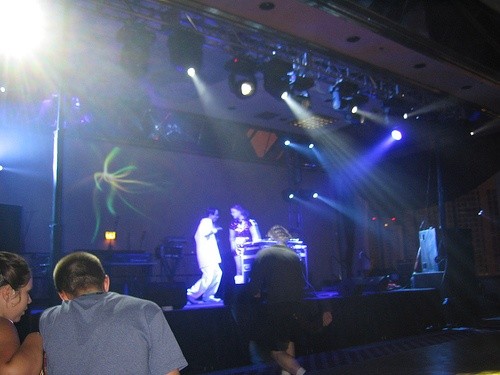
top-left (410, 270), bottom-right (445, 289)
top-left (132, 281), bottom-right (187, 310)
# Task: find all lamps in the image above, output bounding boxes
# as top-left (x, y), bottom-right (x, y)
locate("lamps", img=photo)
top-left (330, 78), bottom-right (360, 114)
top-left (263, 59), bottom-right (292, 101)
top-left (163, 19), bottom-right (204, 76)
top-left (224, 47), bottom-right (257, 99)
top-left (292, 71), bottom-right (314, 98)
top-left (382, 96), bottom-right (415, 142)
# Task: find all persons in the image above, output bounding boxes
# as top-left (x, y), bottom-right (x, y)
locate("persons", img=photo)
top-left (186, 207), bottom-right (223, 304)
top-left (39, 252), bottom-right (188, 375)
top-left (0, 251), bottom-right (44, 375)
top-left (229, 225), bottom-right (333, 375)
top-left (228, 205), bottom-right (261, 275)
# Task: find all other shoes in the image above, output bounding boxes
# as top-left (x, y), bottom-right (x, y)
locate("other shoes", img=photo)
top-left (303, 371), bottom-right (311, 375)
top-left (187, 296), bottom-right (198, 304)
top-left (203, 295), bottom-right (221, 302)
